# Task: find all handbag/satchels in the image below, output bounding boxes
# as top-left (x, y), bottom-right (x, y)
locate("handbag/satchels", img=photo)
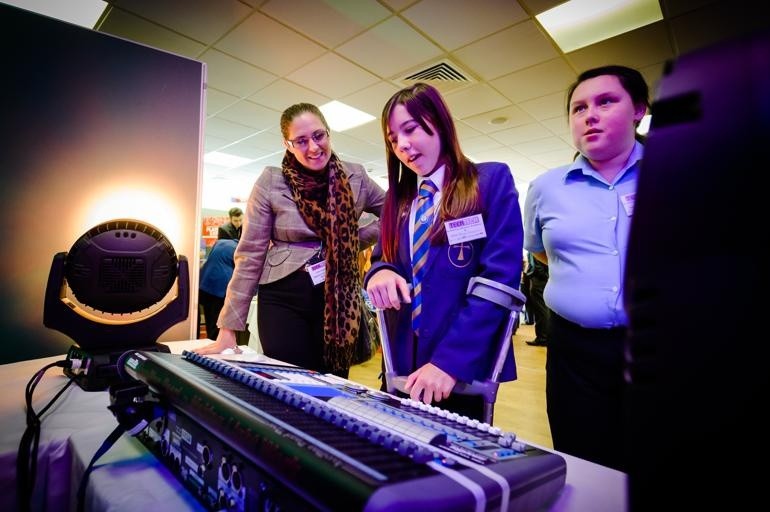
top-left (351, 290), bottom-right (381, 366)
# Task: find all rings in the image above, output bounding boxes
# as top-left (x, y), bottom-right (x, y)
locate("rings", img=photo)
top-left (233, 345), bottom-right (239, 350)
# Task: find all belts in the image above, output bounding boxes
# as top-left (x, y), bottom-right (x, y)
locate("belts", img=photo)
top-left (300, 265), bottom-right (313, 272)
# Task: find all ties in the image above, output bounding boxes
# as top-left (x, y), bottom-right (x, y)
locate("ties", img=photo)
top-left (411, 180), bottom-right (438, 338)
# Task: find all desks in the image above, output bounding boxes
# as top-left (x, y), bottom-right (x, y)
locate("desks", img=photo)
top-left (67, 428), bottom-right (626, 512)
top-left (0, 339), bottom-right (261, 511)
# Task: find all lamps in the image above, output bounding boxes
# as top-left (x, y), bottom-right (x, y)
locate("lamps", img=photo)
top-left (43, 218), bottom-right (189, 392)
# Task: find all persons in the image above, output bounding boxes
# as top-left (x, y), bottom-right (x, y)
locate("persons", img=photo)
top-left (189, 102), bottom-right (391, 381)
top-left (217, 207), bottom-right (247, 242)
top-left (526, 250), bottom-right (553, 347)
top-left (524, 66), bottom-right (651, 474)
top-left (198, 227), bottom-right (249, 345)
top-left (521, 259), bottom-right (534, 324)
top-left (364, 85), bottom-right (524, 427)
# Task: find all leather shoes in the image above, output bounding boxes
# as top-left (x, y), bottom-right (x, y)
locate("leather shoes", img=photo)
top-left (526, 337), bottom-right (547, 346)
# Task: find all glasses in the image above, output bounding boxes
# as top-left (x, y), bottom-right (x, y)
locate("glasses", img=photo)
top-left (287, 132), bottom-right (328, 149)
